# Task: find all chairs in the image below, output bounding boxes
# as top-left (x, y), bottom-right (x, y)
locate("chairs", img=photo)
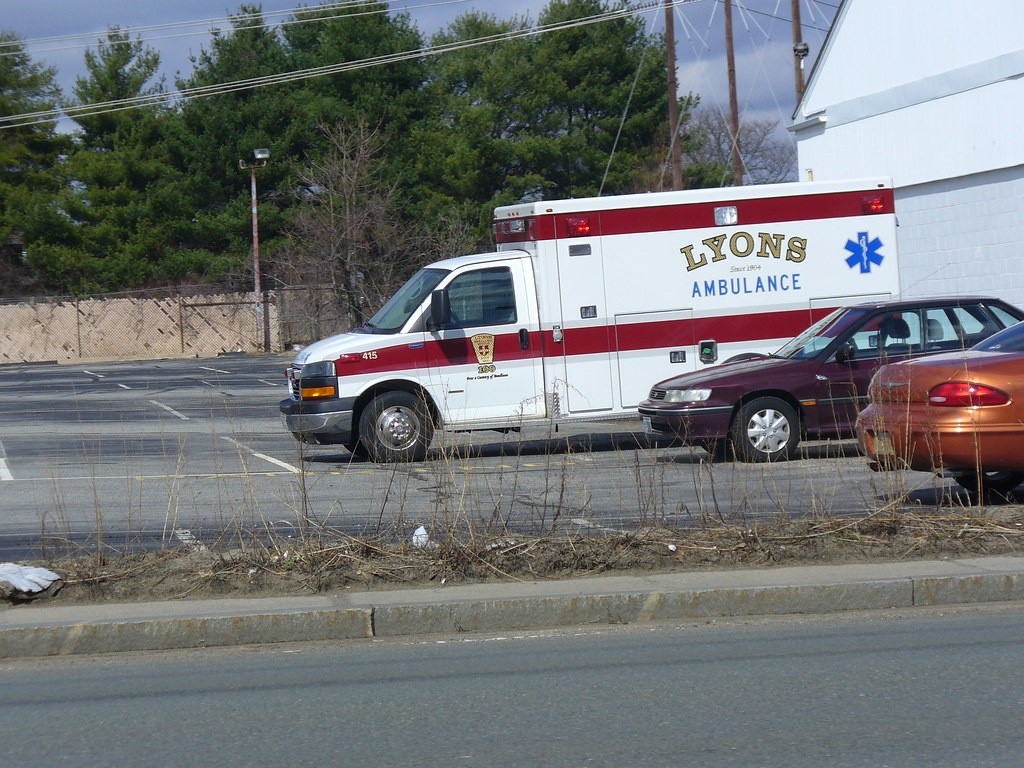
top-left (925, 319), bottom-right (943, 349)
top-left (884, 319), bottom-right (912, 355)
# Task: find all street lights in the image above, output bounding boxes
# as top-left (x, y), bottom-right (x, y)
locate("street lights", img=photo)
top-left (237, 145), bottom-right (273, 352)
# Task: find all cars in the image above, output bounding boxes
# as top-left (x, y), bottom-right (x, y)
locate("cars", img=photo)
top-left (636, 295), bottom-right (1024, 464)
top-left (853, 317), bottom-right (1024, 501)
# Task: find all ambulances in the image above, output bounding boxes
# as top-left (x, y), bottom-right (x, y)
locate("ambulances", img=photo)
top-left (277, 176), bottom-right (903, 464)
